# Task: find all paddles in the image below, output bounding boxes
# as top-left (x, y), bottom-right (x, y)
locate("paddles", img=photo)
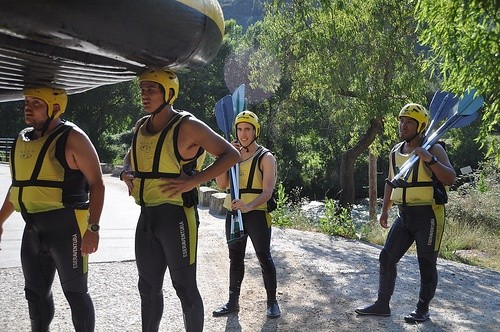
top-left (214, 84), bottom-right (247, 244)
top-left (387, 85), bottom-right (485, 190)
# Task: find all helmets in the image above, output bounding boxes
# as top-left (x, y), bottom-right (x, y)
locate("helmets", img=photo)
top-left (399, 104), bottom-right (428, 134)
top-left (235, 111), bottom-right (260, 139)
top-left (139, 68), bottom-right (179, 104)
top-left (23, 86), bottom-right (67, 120)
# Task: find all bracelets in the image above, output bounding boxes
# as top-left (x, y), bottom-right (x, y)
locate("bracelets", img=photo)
top-left (120, 170), bottom-right (128, 181)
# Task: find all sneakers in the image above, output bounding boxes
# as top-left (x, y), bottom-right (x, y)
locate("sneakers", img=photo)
top-left (213, 302), bottom-right (240, 316)
top-left (267, 300), bottom-right (280, 319)
top-left (405, 308), bottom-right (430, 321)
top-left (355, 301), bottom-right (391, 316)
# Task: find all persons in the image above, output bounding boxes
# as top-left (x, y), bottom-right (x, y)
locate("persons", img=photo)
top-left (354, 103), bottom-right (456, 323)
top-left (213, 111), bottom-right (281, 319)
top-left (119, 67), bottom-right (243, 332)
top-left (0, 86), bottom-right (104, 332)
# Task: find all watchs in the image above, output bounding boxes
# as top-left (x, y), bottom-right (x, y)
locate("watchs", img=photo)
top-left (87, 223), bottom-right (100, 233)
